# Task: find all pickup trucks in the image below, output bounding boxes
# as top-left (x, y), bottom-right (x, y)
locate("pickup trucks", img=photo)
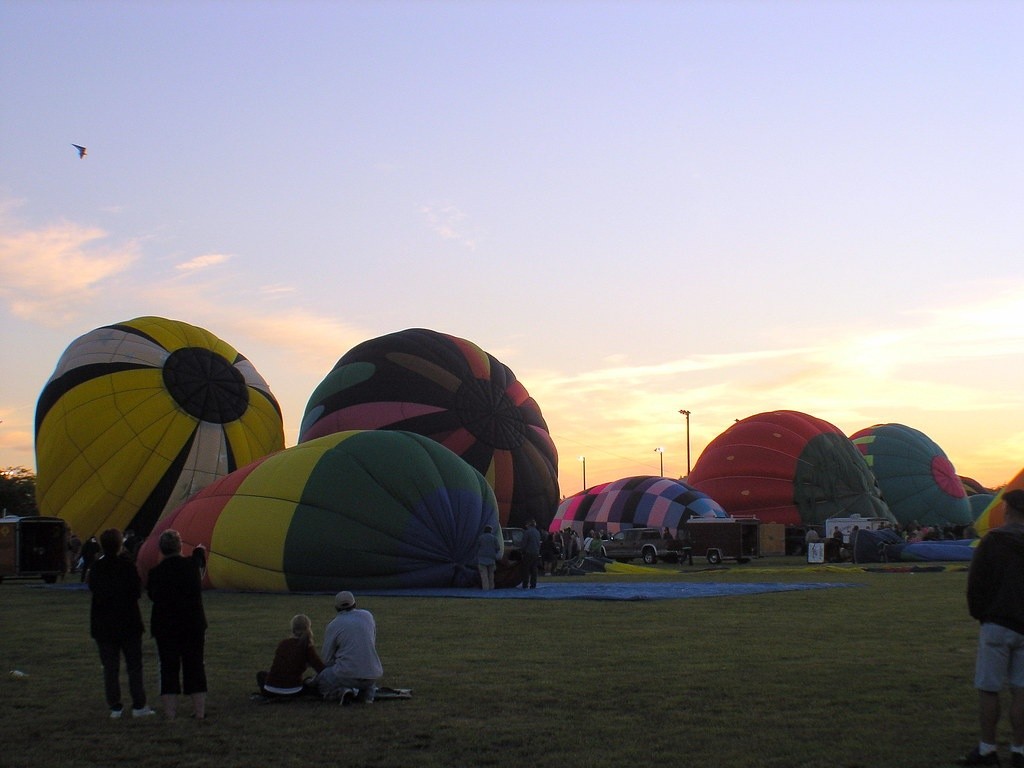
top-left (597, 527), bottom-right (694, 564)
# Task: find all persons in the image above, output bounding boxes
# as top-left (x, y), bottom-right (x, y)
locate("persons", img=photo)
top-left (679, 531), bottom-right (693, 567)
top-left (256, 614), bottom-right (328, 705)
top-left (473, 525), bottom-right (500, 592)
top-left (87, 528), bottom-right (155, 718)
top-left (951, 489), bottom-right (1024, 768)
top-left (539, 525), bottom-right (614, 576)
top-left (147, 529), bottom-right (208, 722)
top-left (520, 521), bottom-right (541, 589)
top-left (663, 527), bottom-right (673, 540)
top-left (68, 530), bottom-right (143, 582)
top-left (306, 590), bottom-right (384, 707)
top-left (803, 520), bottom-right (981, 564)
top-left (191, 543), bottom-right (206, 581)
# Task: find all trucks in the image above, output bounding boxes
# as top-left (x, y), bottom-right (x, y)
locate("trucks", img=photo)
top-left (0, 513), bottom-right (69, 584)
top-left (672, 514), bottom-right (763, 565)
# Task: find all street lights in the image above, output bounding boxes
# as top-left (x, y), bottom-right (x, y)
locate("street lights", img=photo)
top-left (678, 409), bottom-right (691, 476)
top-left (653, 447), bottom-right (665, 480)
top-left (578, 456), bottom-right (588, 489)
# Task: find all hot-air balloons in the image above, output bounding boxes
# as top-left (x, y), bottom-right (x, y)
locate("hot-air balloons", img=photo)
top-left (550, 475), bottom-right (730, 542)
top-left (958, 474), bottom-right (988, 497)
top-left (968, 493), bottom-right (999, 519)
top-left (967, 467), bottom-right (1024, 542)
top-left (846, 421), bottom-right (974, 528)
top-left (295, 326), bottom-right (561, 533)
top-left (135, 429), bottom-right (506, 590)
top-left (685, 409), bottom-right (899, 544)
top-left (34, 316), bottom-right (286, 573)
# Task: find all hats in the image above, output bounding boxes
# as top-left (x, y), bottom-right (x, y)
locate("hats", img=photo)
top-left (335, 591), bottom-right (357, 609)
top-left (1000, 489), bottom-right (1024, 512)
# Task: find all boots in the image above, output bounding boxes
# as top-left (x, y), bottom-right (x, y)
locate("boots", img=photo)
top-left (161, 692), bottom-right (178, 720)
top-left (189, 692), bottom-right (205, 719)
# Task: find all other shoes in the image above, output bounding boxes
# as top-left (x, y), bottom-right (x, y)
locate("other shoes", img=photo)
top-left (957, 746), bottom-right (1023, 768)
top-left (340, 686), bottom-right (375, 706)
top-left (109, 707), bottom-right (157, 719)
